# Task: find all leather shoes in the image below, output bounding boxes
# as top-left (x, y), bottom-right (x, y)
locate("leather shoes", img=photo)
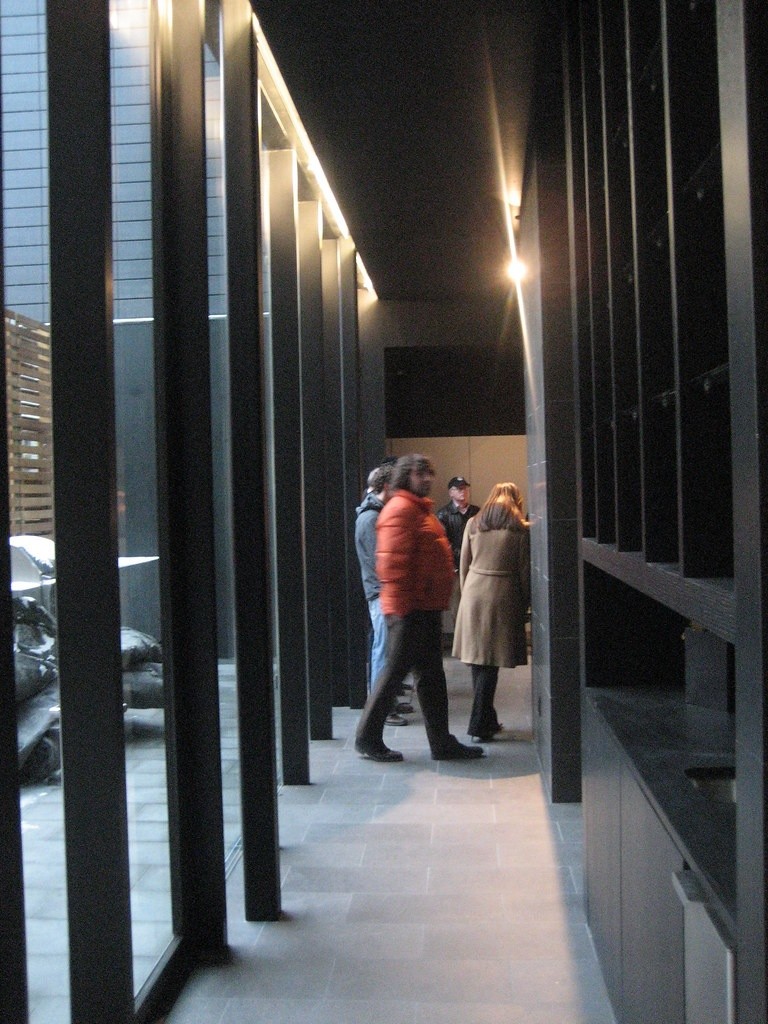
top-left (384, 714), bottom-right (408, 726)
top-left (432, 742), bottom-right (483, 761)
top-left (396, 701), bottom-right (414, 714)
top-left (355, 740), bottom-right (403, 762)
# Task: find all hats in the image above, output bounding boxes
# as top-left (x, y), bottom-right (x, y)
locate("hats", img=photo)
top-left (366, 467), bottom-right (381, 494)
top-left (448, 477), bottom-right (470, 490)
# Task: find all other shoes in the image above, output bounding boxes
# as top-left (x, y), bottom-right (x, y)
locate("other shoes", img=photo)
top-left (395, 683), bottom-right (412, 696)
top-left (472, 735), bottom-right (488, 744)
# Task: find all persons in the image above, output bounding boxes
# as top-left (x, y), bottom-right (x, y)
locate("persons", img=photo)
top-left (452, 483), bottom-right (531, 741)
top-left (355, 457), bottom-right (415, 725)
top-left (355, 454), bottom-right (484, 763)
top-left (435, 477), bottom-right (480, 626)
